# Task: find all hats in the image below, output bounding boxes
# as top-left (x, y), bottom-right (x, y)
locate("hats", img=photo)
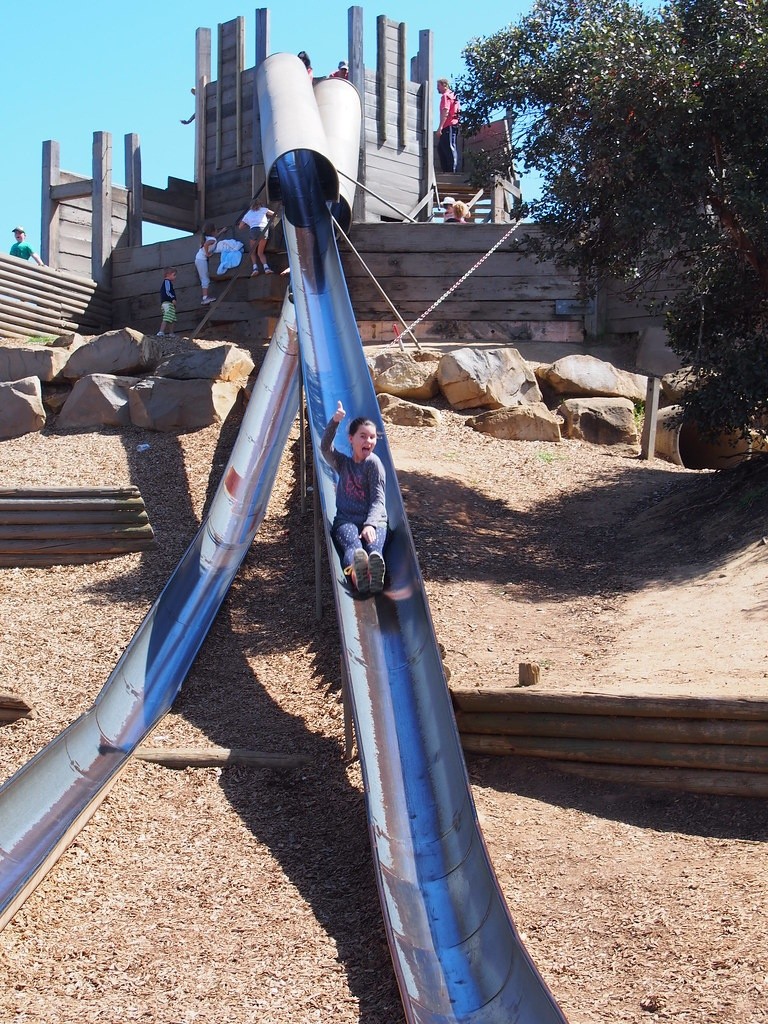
top-left (440, 197), bottom-right (456, 204)
top-left (11, 225), bottom-right (25, 235)
top-left (338, 61), bottom-right (347, 70)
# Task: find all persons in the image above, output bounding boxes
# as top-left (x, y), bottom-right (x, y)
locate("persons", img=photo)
top-left (319, 400), bottom-right (392, 597)
top-left (9, 228), bottom-right (50, 268)
top-left (436, 80), bottom-right (459, 173)
top-left (157, 266), bottom-right (178, 338)
top-left (213, 229), bottom-right (245, 282)
top-left (330, 62), bottom-right (348, 79)
top-left (239, 197), bottom-right (278, 277)
top-left (441, 197), bottom-right (470, 224)
top-left (194, 223), bottom-right (228, 303)
top-left (298, 51), bottom-right (313, 82)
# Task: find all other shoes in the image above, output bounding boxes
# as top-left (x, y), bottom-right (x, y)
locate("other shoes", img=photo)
top-left (367, 551), bottom-right (385, 593)
top-left (156, 331), bottom-right (165, 337)
top-left (251, 268), bottom-right (260, 277)
top-left (169, 333), bottom-right (176, 338)
top-left (200, 296), bottom-right (217, 305)
top-left (264, 267), bottom-right (275, 275)
top-left (344, 547), bottom-right (369, 593)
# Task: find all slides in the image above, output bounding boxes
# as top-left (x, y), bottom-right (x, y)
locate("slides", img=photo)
top-left (279, 199), bottom-right (572, 1024)
top-left (0, 278), bottom-right (306, 934)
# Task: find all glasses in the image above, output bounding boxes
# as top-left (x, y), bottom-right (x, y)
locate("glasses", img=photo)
top-left (299, 57), bottom-right (306, 61)
top-left (341, 68), bottom-right (347, 72)
